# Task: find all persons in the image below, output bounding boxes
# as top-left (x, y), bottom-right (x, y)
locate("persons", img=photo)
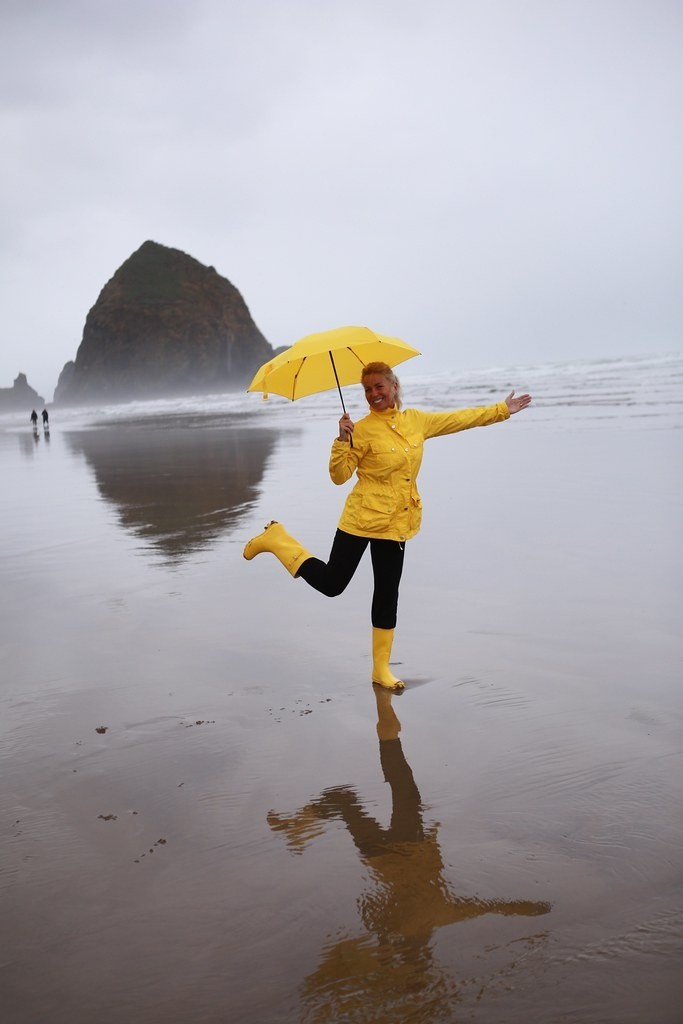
top-left (41, 409), bottom-right (49, 426)
top-left (243, 362), bottom-right (532, 692)
top-left (30, 409), bottom-right (38, 425)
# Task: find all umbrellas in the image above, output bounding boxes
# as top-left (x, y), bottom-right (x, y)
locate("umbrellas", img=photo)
top-left (245, 325), bottom-right (422, 448)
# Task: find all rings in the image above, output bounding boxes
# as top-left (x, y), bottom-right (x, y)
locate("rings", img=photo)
top-left (520, 403), bottom-right (523, 407)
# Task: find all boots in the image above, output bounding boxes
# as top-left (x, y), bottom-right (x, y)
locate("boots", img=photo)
top-left (243, 520), bottom-right (315, 580)
top-left (372, 626), bottom-right (405, 689)
top-left (372, 682), bottom-right (401, 734)
